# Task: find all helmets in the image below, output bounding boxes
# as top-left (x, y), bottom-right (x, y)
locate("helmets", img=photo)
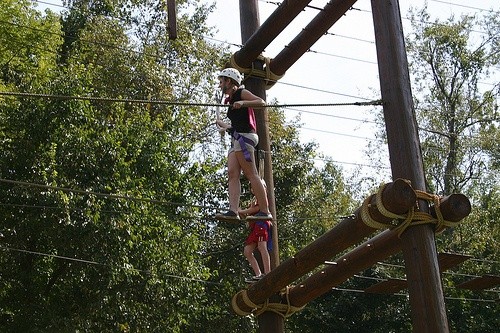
top-left (248, 177), bottom-right (266, 188)
top-left (218, 68), bottom-right (245, 85)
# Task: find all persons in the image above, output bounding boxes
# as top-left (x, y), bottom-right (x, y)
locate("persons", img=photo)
top-left (215, 68), bottom-right (273, 220)
top-left (238, 179), bottom-right (274, 283)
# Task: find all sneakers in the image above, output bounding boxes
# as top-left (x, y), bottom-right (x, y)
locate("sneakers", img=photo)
top-left (254, 274), bottom-right (267, 280)
top-left (216, 210), bottom-right (240, 221)
top-left (246, 212), bottom-right (273, 220)
top-left (246, 278), bottom-right (258, 283)
